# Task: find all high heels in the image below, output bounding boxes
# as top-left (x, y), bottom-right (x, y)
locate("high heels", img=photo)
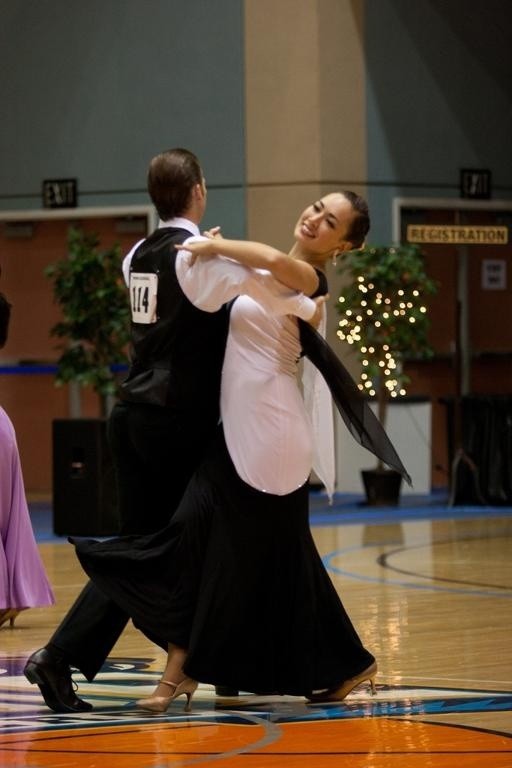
top-left (303, 658), bottom-right (378, 706)
top-left (135, 676), bottom-right (199, 713)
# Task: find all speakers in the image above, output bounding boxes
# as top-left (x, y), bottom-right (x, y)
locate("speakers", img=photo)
top-left (448, 394), bottom-right (511, 506)
top-left (50, 415), bottom-right (112, 540)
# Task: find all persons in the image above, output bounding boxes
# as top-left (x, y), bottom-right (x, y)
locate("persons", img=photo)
top-left (0, 407), bottom-right (55, 628)
top-left (24, 148), bottom-right (329, 713)
top-left (135, 190), bottom-right (377, 716)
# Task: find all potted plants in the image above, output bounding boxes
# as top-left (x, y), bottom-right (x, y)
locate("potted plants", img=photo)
top-left (330, 244), bottom-right (447, 506)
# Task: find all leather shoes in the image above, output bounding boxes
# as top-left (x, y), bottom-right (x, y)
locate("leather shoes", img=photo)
top-left (22, 647), bottom-right (94, 713)
